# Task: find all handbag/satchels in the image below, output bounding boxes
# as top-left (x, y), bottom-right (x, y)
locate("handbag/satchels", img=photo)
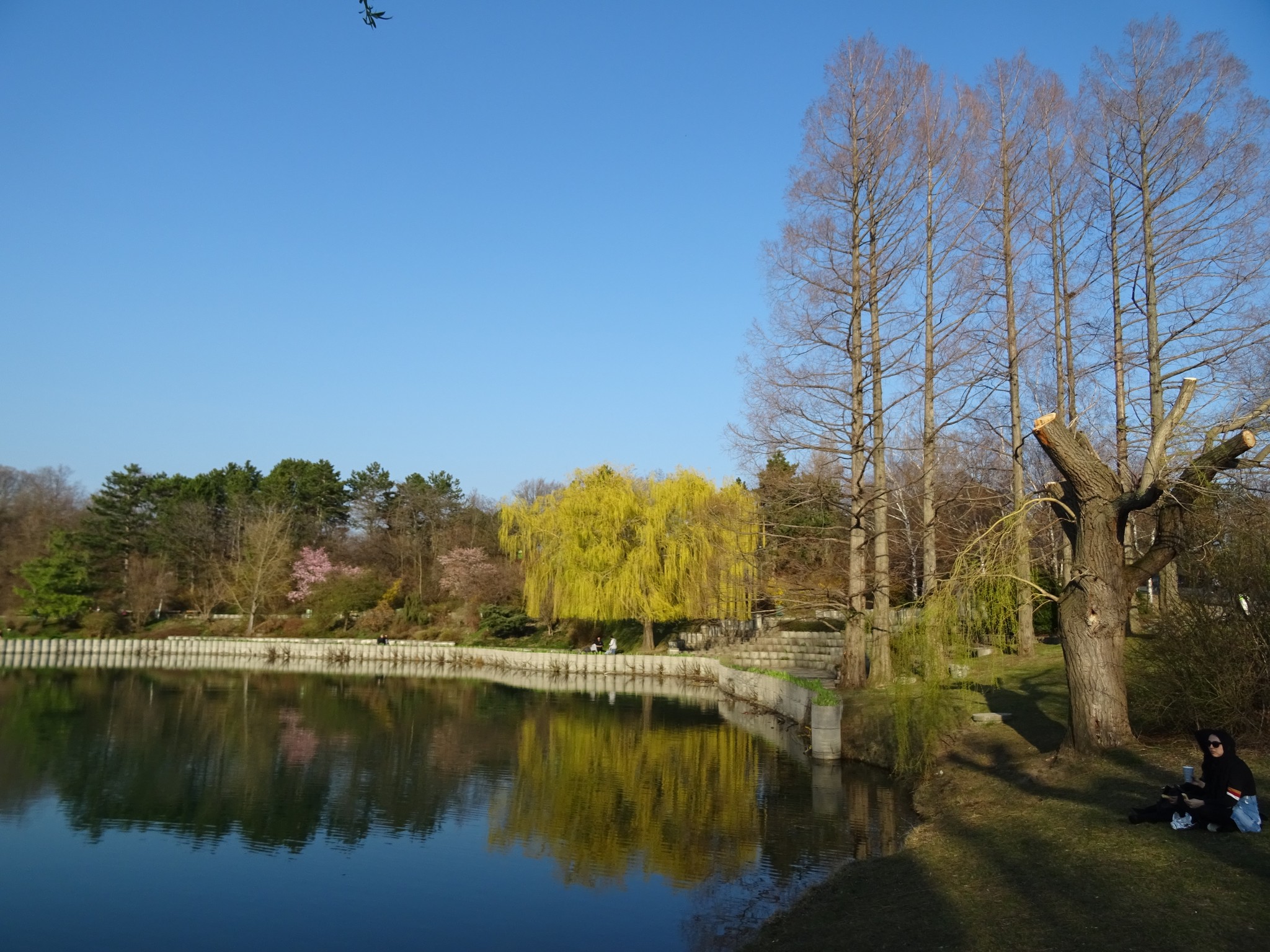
top-left (1230, 795), bottom-right (1261, 833)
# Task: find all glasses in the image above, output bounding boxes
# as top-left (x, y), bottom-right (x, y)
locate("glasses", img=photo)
top-left (1206, 739), bottom-right (1222, 748)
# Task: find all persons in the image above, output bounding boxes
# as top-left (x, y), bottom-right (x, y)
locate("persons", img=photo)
top-left (595, 637), bottom-right (604, 652)
top-left (1228, 594), bottom-right (1250, 616)
top-left (385, 634), bottom-right (388, 644)
top-left (377, 634), bottom-right (386, 645)
top-left (590, 641), bottom-right (598, 652)
top-left (609, 636), bottom-right (617, 655)
top-left (1177, 729), bottom-right (1261, 833)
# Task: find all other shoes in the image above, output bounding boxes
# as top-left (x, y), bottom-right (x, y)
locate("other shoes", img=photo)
top-left (1207, 823), bottom-right (1220, 833)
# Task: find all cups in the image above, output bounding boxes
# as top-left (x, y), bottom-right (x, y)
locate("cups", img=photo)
top-left (1182, 766), bottom-right (1194, 782)
